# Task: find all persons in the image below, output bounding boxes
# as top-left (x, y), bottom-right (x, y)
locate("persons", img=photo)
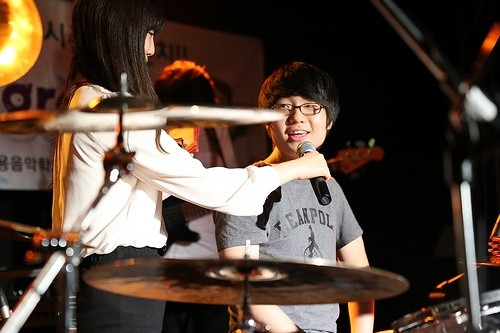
top-left (52, 0), bottom-right (331, 333)
top-left (152, 59), bottom-right (238, 333)
top-left (214, 62), bottom-right (375, 333)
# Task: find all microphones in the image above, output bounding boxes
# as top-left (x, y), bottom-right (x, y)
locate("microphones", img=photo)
top-left (296, 141), bottom-right (332, 206)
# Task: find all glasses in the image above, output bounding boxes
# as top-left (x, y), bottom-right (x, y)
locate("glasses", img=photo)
top-left (271, 103), bottom-right (324, 116)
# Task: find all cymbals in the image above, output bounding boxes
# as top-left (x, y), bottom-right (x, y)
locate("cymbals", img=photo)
top-left (42, 92), bottom-right (284, 133)
top-left (435, 262), bottom-right (500, 294)
top-left (0, 0), bottom-right (45, 88)
top-left (83, 254), bottom-right (409, 306)
